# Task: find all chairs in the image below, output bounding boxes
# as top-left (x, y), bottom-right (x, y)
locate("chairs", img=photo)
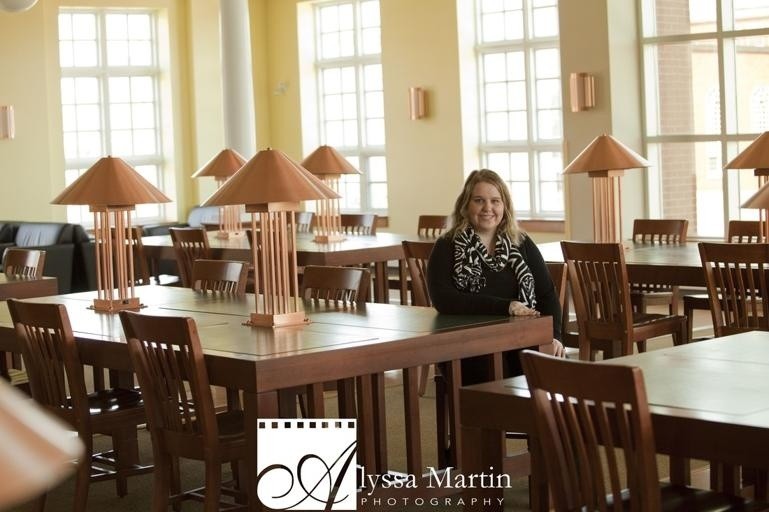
top-left (1, 207), bottom-right (769, 512)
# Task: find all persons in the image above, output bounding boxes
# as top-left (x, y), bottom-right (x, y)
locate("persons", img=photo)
top-left (426, 168), bottom-right (570, 469)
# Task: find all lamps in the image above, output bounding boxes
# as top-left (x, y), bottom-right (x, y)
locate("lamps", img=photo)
top-left (570, 72), bottom-right (595, 112)
top-left (408, 87), bottom-right (429, 120)
top-left (49, 146), bottom-right (364, 330)
top-left (562, 134), bottom-right (651, 250)
top-left (0, 105), bottom-right (15, 138)
top-left (724, 131), bottom-right (769, 244)
